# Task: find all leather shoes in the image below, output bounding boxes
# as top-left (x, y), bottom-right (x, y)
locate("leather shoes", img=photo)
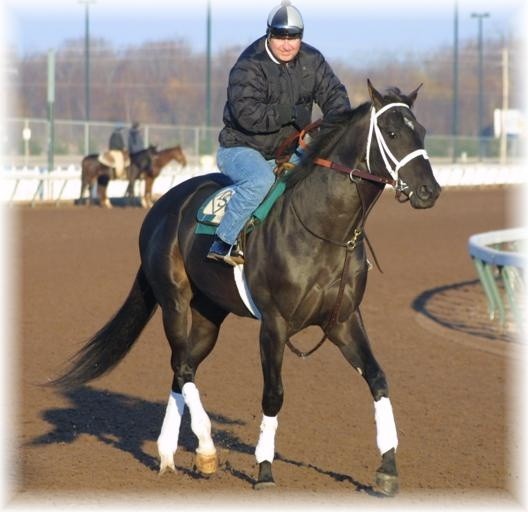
top-left (206, 238), bottom-right (246, 265)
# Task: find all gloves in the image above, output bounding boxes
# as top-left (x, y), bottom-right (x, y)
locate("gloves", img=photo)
top-left (291, 106), bottom-right (312, 131)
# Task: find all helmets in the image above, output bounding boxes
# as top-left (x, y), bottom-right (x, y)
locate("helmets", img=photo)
top-left (267, 0), bottom-right (304, 38)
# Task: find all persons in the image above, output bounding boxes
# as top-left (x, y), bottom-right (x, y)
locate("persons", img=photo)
top-left (127, 116), bottom-right (152, 183)
top-left (107, 123), bottom-right (127, 153)
top-left (204, 2), bottom-right (351, 265)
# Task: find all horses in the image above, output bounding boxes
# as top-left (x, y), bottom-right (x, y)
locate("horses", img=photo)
top-left (34, 75), bottom-right (443, 498)
top-left (78, 140), bottom-right (188, 210)
